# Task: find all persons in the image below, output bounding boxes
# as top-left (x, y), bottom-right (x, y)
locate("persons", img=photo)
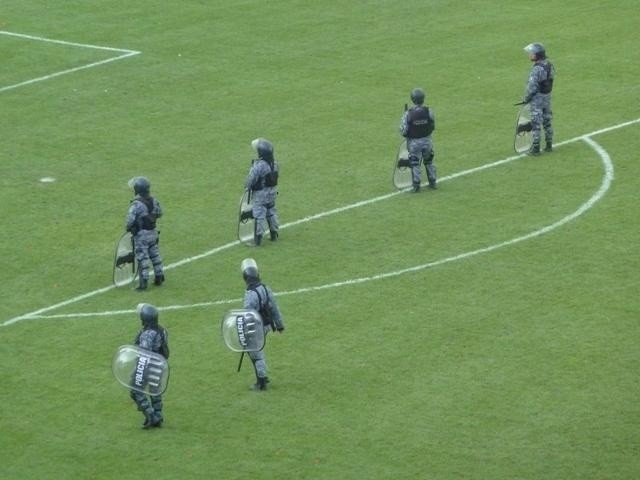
top-left (522, 42), bottom-right (556, 157)
top-left (127, 302), bottom-right (170, 429)
top-left (123, 175), bottom-right (166, 291)
top-left (244, 136), bottom-right (280, 248)
top-left (400, 87), bottom-right (438, 193)
top-left (240, 257), bottom-right (286, 391)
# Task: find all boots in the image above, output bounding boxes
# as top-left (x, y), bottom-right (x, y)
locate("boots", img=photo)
top-left (413, 182), bottom-right (420, 192)
top-left (143, 412), bottom-right (164, 427)
top-left (154, 273), bottom-right (165, 286)
top-left (530, 142), bottom-right (540, 155)
top-left (430, 181), bottom-right (438, 189)
top-left (256, 235), bottom-right (262, 246)
top-left (544, 142), bottom-right (553, 152)
top-left (255, 377), bottom-right (269, 391)
top-left (272, 232), bottom-right (279, 241)
top-left (136, 280), bottom-right (147, 290)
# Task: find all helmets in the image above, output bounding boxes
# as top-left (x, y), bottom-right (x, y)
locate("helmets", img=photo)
top-left (524, 43), bottom-right (546, 60)
top-left (128, 177), bottom-right (151, 194)
top-left (252, 138), bottom-right (273, 157)
top-left (137, 303), bottom-right (159, 325)
top-left (242, 258), bottom-right (259, 284)
top-left (411, 89), bottom-right (424, 104)
